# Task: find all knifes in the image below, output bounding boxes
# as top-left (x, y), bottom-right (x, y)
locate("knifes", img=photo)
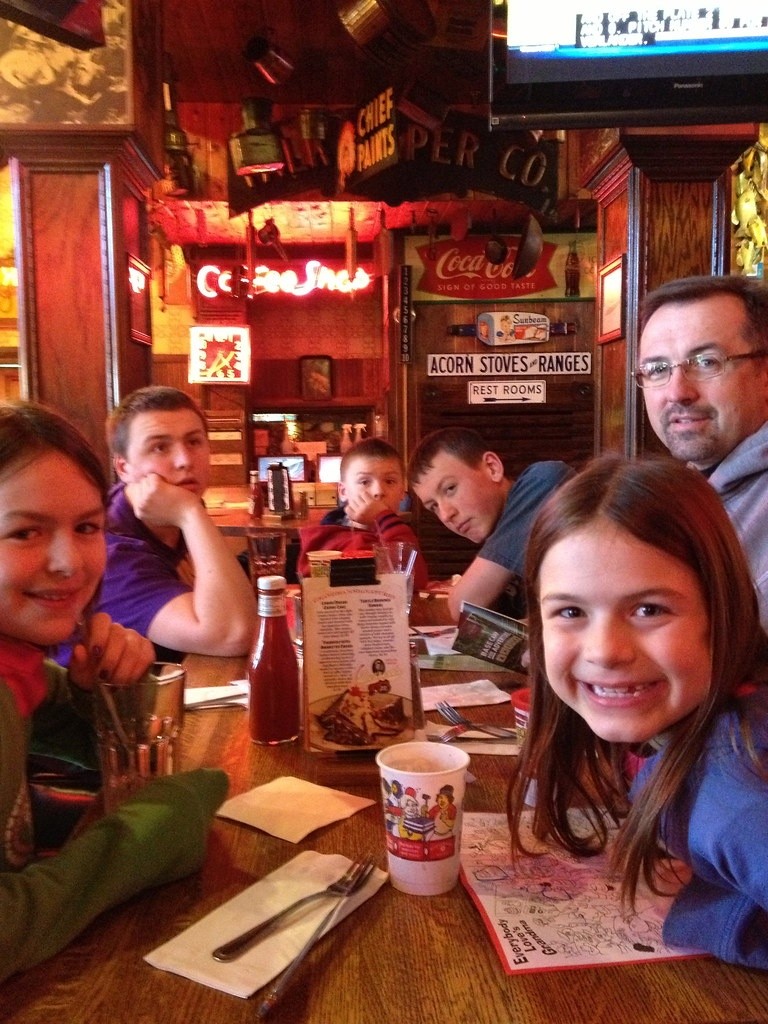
top-left (255, 857), bottom-right (373, 1019)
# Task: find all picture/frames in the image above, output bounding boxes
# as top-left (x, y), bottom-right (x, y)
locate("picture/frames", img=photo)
top-left (127, 251), bottom-right (153, 346)
top-left (597, 253), bottom-right (625, 344)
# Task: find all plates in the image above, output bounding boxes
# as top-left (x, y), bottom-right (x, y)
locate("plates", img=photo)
top-left (310, 691), bottom-right (416, 750)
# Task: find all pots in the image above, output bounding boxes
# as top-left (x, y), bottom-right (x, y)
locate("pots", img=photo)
top-left (485, 207), bottom-right (507, 264)
top-left (511, 214), bottom-right (544, 281)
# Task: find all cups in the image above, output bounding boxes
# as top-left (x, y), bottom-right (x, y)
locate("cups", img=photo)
top-left (292, 590), bottom-right (303, 648)
top-left (372, 541), bottom-right (417, 616)
top-left (306, 550), bottom-right (342, 577)
top-left (91, 660), bottom-right (189, 812)
top-left (512, 687), bottom-right (534, 747)
top-left (374, 740), bottom-right (469, 896)
top-left (246, 530), bottom-right (286, 599)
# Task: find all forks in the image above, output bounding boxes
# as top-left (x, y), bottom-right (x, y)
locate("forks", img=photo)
top-left (429, 700), bottom-right (520, 746)
top-left (212, 847), bottom-right (385, 962)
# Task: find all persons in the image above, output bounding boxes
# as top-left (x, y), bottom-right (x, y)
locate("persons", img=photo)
top-left (48, 386), bottom-right (260, 666)
top-left (506, 456), bottom-right (768, 968)
top-left (297, 437), bottom-right (427, 579)
top-left (407, 427), bottom-right (579, 620)
top-left (0, 405), bottom-right (229, 986)
top-left (521, 275), bottom-right (768, 668)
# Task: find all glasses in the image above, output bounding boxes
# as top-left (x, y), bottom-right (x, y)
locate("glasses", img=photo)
top-left (629, 349), bottom-right (768, 387)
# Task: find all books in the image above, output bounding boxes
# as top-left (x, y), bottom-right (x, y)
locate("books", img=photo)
top-left (453, 601), bottom-right (531, 676)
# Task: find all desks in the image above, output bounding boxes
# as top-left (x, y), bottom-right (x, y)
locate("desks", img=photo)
top-left (201, 486), bottom-right (337, 539)
top-left (0, 585), bottom-right (768, 1024)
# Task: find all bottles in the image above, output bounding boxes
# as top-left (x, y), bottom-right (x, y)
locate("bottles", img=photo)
top-left (248, 575), bottom-right (302, 746)
top-left (247, 469), bottom-right (264, 519)
top-left (566, 240), bottom-right (580, 296)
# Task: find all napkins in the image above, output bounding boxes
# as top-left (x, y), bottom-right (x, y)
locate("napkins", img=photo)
top-left (420, 680), bottom-right (512, 712)
top-left (214, 776), bottom-right (377, 844)
top-left (181, 683), bottom-right (249, 711)
top-left (144, 850), bottom-right (387, 998)
top-left (407, 625), bottom-right (463, 656)
top-left (426, 720), bottom-right (519, 756)
